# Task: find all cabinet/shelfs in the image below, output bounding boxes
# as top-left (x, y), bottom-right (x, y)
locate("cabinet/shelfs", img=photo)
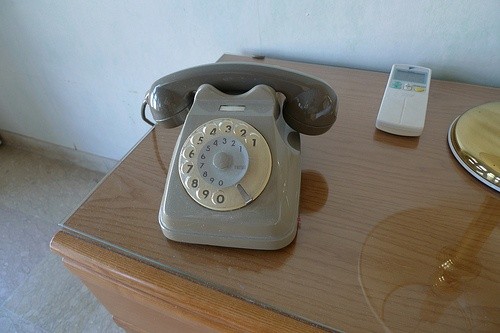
top-left (48, 52), bottom-right (500, 333)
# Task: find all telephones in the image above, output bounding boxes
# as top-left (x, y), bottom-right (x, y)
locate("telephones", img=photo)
top-left (147, 63), bottom-right (338, 251)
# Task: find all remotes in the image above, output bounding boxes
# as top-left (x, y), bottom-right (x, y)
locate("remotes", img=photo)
top-left (375, 64), bottom-right (431, 137)
